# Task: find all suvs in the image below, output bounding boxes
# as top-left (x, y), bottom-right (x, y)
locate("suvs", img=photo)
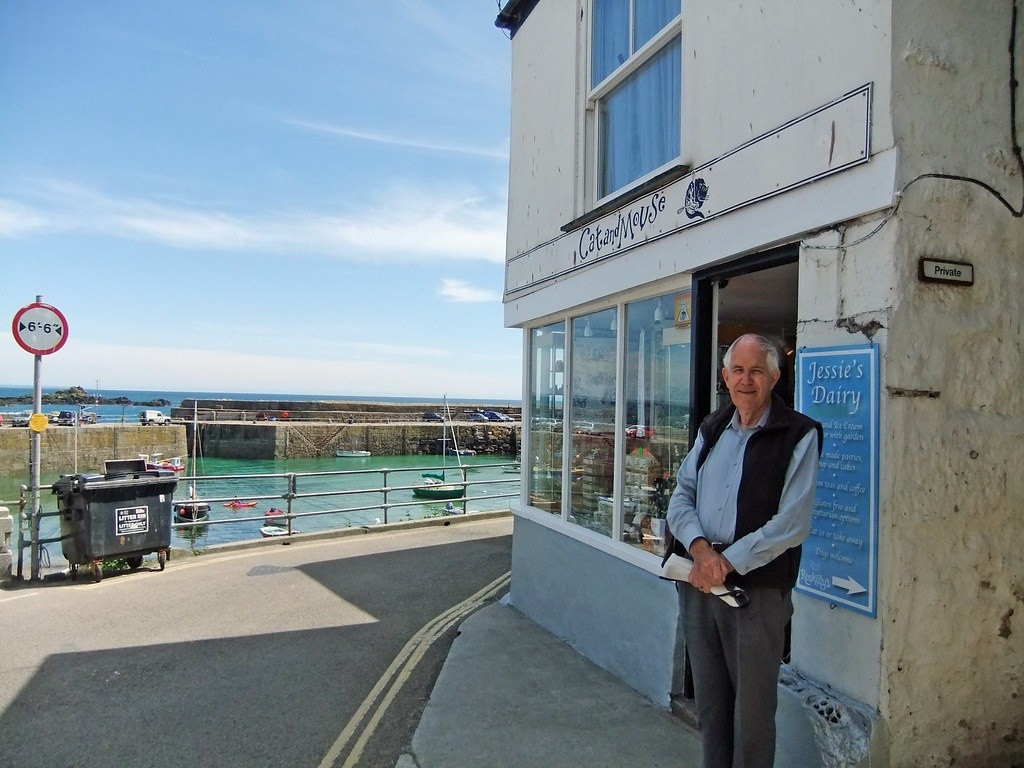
top-left (530, 416), bottom-right (616, 437)
top-left (484, 411), bottom-right (506, 422)
top-left (140, 409), bottom-right (172, 426)
top-left (57, 411), bottom-right (77, 426)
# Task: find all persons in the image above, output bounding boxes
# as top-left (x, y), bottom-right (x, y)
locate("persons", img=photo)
top-left (661, 333), bottom-right (823, 768)
top-left (349, 415), bottom-right (353, 424)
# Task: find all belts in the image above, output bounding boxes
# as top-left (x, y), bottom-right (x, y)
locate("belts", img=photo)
top-left (709, 541), bottom-right (732, 554)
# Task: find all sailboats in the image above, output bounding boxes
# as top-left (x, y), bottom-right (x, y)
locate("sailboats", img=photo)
top-left (412, 393), bottom-right (466, 500)
top-left (171, 400), bottom-right (211, 526)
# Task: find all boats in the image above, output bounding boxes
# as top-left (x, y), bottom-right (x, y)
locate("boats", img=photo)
top-left (441, 502), bottom-right (464, 516)
top-left (258, 526), bottom-right (300, 538)
top-left (222, 500), bottom-right (258, 508)
top-left (335, 448), bottom-right (372, 457)
top-left (502, 447), bottom-right (606, 474)
top-left (137, 452), bottom-right (191, 476)
top-left (446, 425), bottom-right (477, 456)
top-left (265, 509), bottom-right (289, 525)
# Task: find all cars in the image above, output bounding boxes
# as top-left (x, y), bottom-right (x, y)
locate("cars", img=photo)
top-left (12, 412), bottom-right (33, 427)
top-left (269, 416), bottom-right (277, 421)
top-left (500, 414), bottom-right (515, 422)
top-left (421, 412), bottom-right (446, 423)
top-left (468, 412), bottom-right (489, 423)
top-left (625, 424), bottom-right (653, 439)
top-left (673, 416), bottom-right (689, 430)
